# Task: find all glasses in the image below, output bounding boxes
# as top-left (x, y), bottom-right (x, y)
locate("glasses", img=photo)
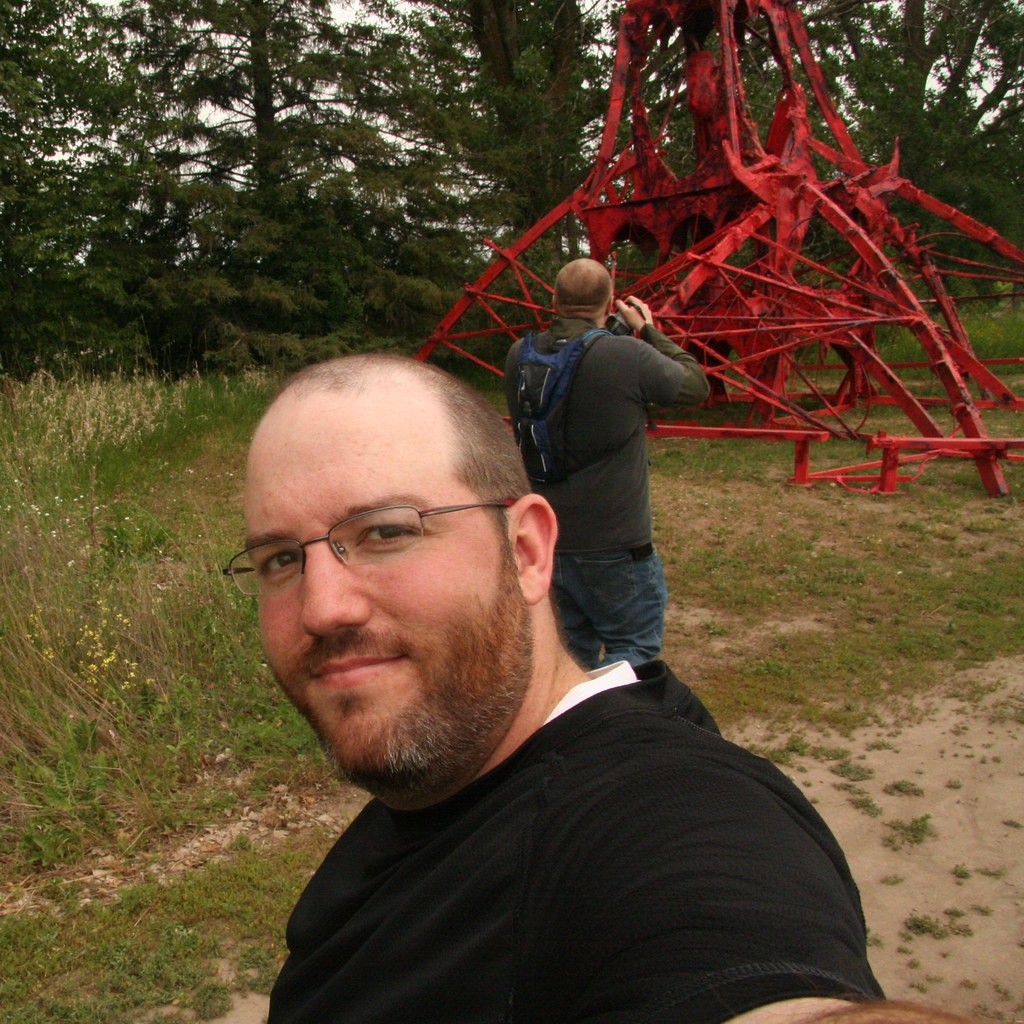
top-left (224, 500), bottom-right (513, 596)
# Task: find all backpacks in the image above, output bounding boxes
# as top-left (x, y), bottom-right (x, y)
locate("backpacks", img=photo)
top-left (513, 325), bottom-right (646, 491)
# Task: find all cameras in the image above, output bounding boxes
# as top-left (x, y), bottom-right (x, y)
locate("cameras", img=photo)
top-left (605, 309), bottom-right (634, 336)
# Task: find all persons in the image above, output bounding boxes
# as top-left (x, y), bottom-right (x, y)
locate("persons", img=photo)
top-left (241, 352), bottom-right (982, 1024)
top-left (501, 257), bottom-right (711, 674)
